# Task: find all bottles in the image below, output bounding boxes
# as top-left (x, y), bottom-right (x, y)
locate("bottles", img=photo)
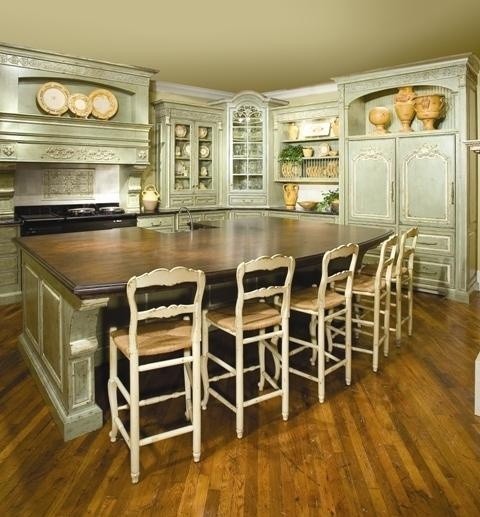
top-left (289, 122), bottom-right (298, 140)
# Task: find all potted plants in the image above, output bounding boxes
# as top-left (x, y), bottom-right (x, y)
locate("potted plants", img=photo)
top-left (314, 191), bottom-right (339, 215)
top-left (277, 144), bottom-right (304, 179)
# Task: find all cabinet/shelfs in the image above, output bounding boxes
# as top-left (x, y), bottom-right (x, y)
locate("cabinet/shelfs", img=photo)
top-left (271, 101), bottom-right (339, 184)
top-left (225, 100), bottom-right (282, 210)
top-left (335, 63), bottom-right (477, 304)
top-left (154, 101), bottom-right (225, 212)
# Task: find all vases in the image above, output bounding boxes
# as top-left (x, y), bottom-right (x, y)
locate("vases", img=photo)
top-left (283, 184), bottom-right (299, 210)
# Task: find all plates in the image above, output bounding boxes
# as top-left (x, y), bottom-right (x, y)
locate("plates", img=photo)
top-left (36, 81), bottom-right (70, 115)
top-left (198, 145), bottom-right (209, 157)
top-left (88, 87), bottom-right (119, 118)
top-left (197, 127), bottom-right (207, 138)
top-left (175, 125), bottom-right (187, 137)
top-left (69, 93), bottom-right (93, 117)
top-left (175, 159), bottom-right (186, 174)
top-left (318, 142), bottom-right (330, 156)
top-left (183, 141), bottom-right (191, 156)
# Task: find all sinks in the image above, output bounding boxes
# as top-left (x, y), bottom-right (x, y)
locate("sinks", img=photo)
top-left (139, 222), bottom-right (221, 235)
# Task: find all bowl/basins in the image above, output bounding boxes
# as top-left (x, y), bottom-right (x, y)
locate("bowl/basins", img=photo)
top-left (297, 200), bottom-right (319, 211)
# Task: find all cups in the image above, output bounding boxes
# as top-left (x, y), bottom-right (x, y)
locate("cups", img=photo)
top-left (302, 148), bottom-right (312, 157)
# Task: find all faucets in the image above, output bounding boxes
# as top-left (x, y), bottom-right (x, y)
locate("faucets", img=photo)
top-left (172, 205), bottom-right (193, 233)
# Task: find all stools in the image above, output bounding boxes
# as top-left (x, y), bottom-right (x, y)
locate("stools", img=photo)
top-left (329, 233), bottom-right (402, 373)
top-left (274, 242), bottom-right (360, 402)
top-left (200, 254), bottom-right (295, 437)
top-left (107, 265), bottom-right (206, 484)
top-left (355, 224), bottom-right (419, 345)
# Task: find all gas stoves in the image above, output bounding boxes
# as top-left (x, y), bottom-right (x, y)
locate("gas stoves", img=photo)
top-left (52, 205), bottom-right (126, 217)
